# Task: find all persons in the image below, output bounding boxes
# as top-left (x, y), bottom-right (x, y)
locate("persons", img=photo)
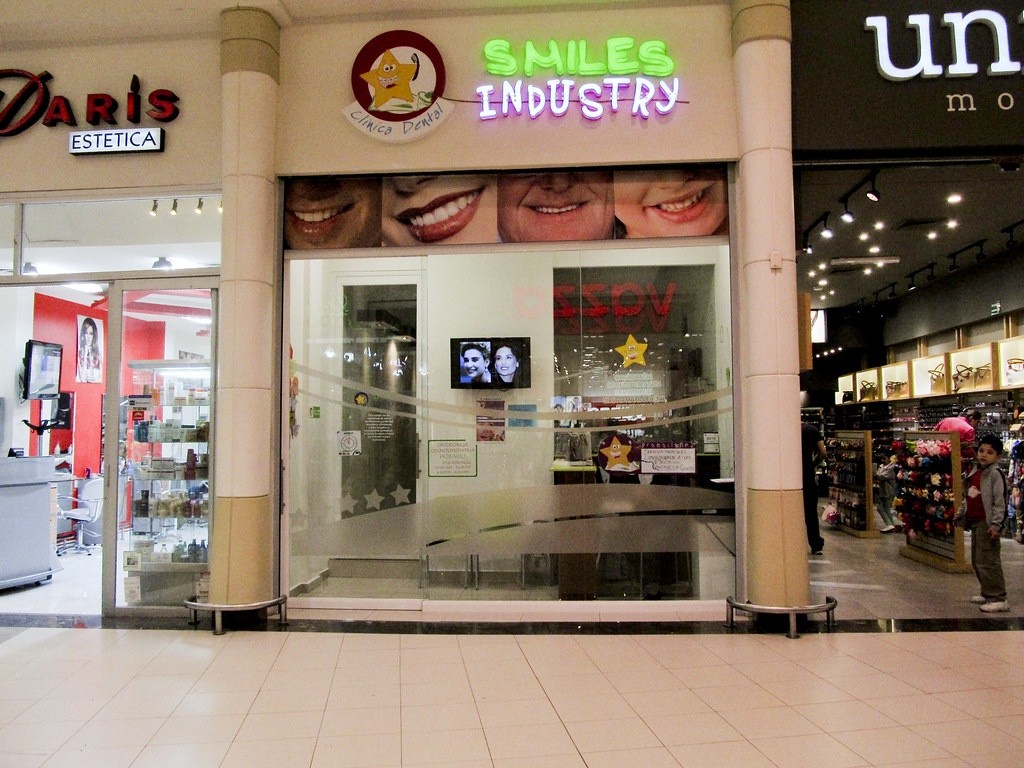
top-left (495, 171), bottom-right (616, 243)
top-left (493, 344), bottom-right (520, 382)
top-left (801, 423), bottom-right (827, 554)
top-left (460, 342), bottom-right (491, 382)
top-left (963, 435), bottom-right (1011, 612)
top-left (78, 317), bottom-right (102, 383)
top-left (616, 170), bottom-right (729, 237)
top-left (285, 176), bottom-right (383, 250)
top-left (555, 397), bottom-right (588, 428)
top-left (876, 453), bottom-right (898, 532)
top-left (383, 175), bottom-right (498, 246)
top-left (932, 408), bottom-right (982, 445)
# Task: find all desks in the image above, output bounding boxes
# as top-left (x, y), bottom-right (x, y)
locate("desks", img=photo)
top-left (551, 458), bottom-right (597, 472)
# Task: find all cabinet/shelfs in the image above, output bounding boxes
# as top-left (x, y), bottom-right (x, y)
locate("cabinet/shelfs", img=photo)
top-left (125, 360), bottom-right (210, 603)
top-left (800, 387), bottom-right (1024, 573)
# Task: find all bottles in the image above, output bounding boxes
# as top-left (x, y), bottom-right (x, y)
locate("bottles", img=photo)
top-left (184, 500), bottom-right (208, 518)
top-left (136, 415), bottom-right (181, 442)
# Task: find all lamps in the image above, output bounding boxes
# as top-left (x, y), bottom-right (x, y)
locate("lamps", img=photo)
top-left (947, 252), bottom-right (960, 273)
top-left (866, 176), bottom-right (880, 202)
top-left (831, 256), bottom-right (901, 264)
top-left (858, 282), bottom-right (897, 313)
top-left (840, 198), bottom-right (854, 224)
top-left (152, 257), bottom-right (171, 269)
top-left (975, 239), bottom-right (988, 263)
top-left (820, 213), bottom-right (832, 240)
top-left (23, 263), bottom-right (38, 275)
top-left (927, 262), bottom-right (936, 282)
top-left (149, 197), bottom-right (223, 216)
top-left (802, 234), bottom-right (813, 254)
top-left (904, 272), bottom-right (916, 291)
top-left (1006, 231), bottom-right (1018, 247)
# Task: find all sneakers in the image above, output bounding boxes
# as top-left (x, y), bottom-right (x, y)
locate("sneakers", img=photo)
top-left (880, 525), bottom-right (894, 532)
top-left (812, 548), bottom-right (823, 555)
top-left (971, 595), bottom-right (985, 603)
top-left (979, 600), bottom-right (1009, 612)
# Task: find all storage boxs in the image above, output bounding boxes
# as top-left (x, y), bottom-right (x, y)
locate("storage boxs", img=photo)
top-left (144, 456), bottom-right (184, 479)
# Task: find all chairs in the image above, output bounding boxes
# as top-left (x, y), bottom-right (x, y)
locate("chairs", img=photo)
top-left (56, 479), bottom-right (103, 556)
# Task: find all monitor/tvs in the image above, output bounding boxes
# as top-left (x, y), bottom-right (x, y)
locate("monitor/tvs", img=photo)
top-left (449, 338), bottom-right (531, 388)
top-left (8, 448), bottom-right (24, 458)
top-left (22, 340), bottom-right (63, 400)
top-left (41, 392), bottom-right (75, 429)
